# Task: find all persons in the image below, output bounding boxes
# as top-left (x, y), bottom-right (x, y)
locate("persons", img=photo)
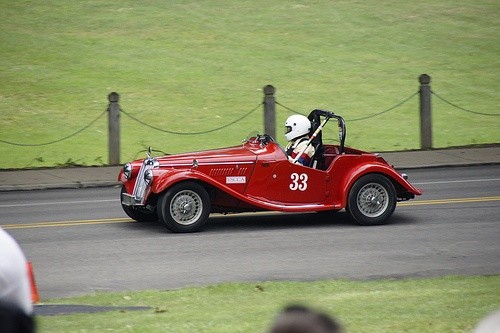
top-left (284, 115), bottom-right (315, 167)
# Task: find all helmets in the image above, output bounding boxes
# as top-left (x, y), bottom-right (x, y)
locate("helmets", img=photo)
top-left (284, 114), bottom-right (311, 141)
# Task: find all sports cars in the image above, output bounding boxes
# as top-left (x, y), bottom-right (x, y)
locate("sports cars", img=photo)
top-left (115, 109), bottom-right (424, 234)
